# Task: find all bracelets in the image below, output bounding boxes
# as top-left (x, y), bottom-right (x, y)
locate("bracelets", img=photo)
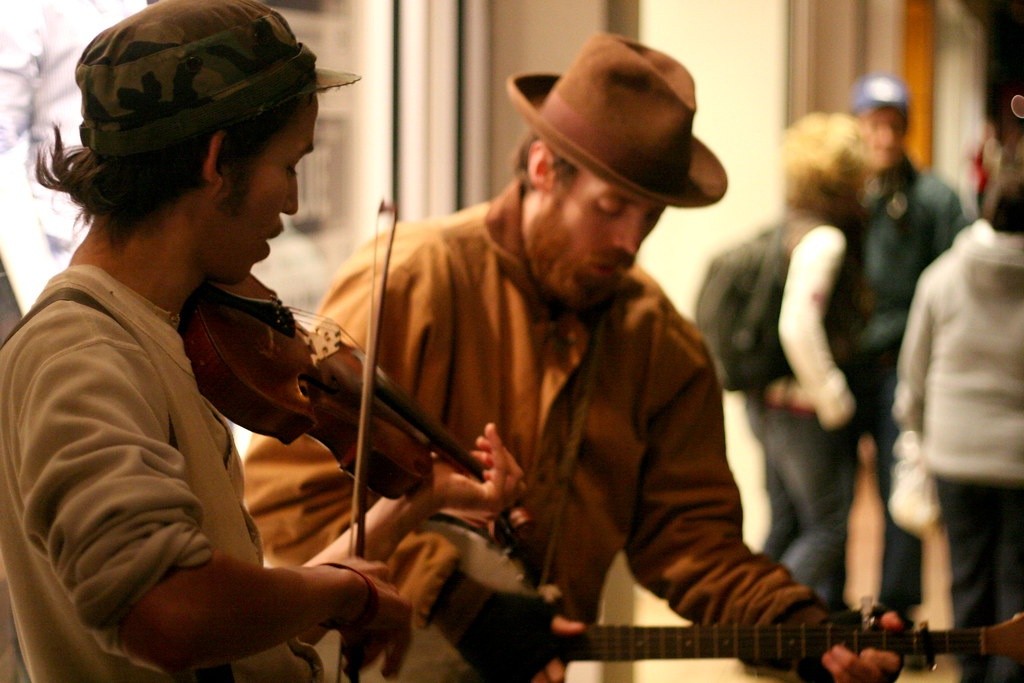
top-left (317, 562), bottom-right (380, 631)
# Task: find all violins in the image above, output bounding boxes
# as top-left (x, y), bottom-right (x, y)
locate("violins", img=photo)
top-left (177, 271), bottom-right (549, 560)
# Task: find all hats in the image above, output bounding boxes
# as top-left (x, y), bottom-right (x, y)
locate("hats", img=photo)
top-left (76, 0), bottom-right (364, 158)
top-left (856, 78), bottom-right (913, 131)
top-left (506, 31), bottom-right (728, 207)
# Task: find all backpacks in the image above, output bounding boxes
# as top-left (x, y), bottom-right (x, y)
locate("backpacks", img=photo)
top-left (695, 216), bottom-right (850, 392)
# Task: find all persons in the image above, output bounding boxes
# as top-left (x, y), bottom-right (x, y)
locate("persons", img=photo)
top-left (0, 0), bottom-right (527, 683)
top-left (234, 30), bottom-right (907, 683)
top-left (740, 75), bottom-right (1024, 683)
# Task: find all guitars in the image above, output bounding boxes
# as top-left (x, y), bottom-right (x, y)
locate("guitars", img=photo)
top-left (304, 511), bottom-right (1024, 683)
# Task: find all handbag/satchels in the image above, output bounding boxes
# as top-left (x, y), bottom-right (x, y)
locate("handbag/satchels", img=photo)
top-left (887, 422), bottom-right (947, 536)
